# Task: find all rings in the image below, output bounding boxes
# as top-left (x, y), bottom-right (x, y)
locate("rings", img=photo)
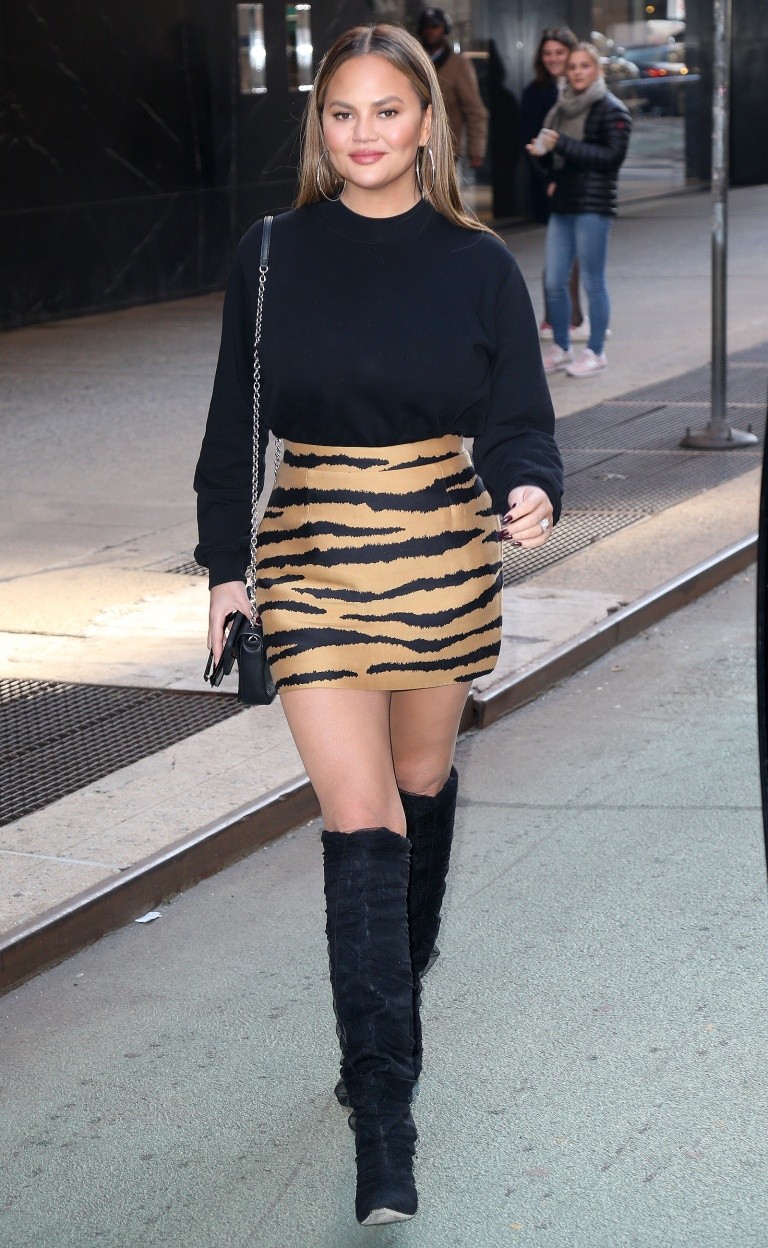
top-left (539, 519), bottom-right (550, 532)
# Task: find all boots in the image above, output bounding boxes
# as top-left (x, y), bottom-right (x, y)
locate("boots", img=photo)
top-left (322, 826), bottom-right (417, 1225)
top-left (335, 764), bottom-right (458, 1107)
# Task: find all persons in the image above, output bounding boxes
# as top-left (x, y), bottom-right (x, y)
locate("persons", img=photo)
top-left (194, 25), bottom-right (564, 1225)
top-left (415, 7), bottom-right (490, 182)
top-left (517, 31), bottom-right (633, 377)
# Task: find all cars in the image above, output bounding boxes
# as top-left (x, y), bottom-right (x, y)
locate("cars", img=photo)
top-left (590, 18), bottom-right (690, 118)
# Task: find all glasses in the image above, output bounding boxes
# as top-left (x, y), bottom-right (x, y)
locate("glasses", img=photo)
top-left (203, 613), bottom-right (245, 687)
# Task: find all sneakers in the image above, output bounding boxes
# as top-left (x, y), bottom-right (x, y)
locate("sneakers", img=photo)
top-left (540, 322), bottom-right (554, 340)
top-left (542, 344), bottom-right (573, 375)
top-left (569, 315), bottom-right (613, 341)
top-left (566, 348), bottom-right (610, 377)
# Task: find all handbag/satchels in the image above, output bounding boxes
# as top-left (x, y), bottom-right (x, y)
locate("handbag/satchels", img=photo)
top-left (235, 619), bottom-right (276, 705)
